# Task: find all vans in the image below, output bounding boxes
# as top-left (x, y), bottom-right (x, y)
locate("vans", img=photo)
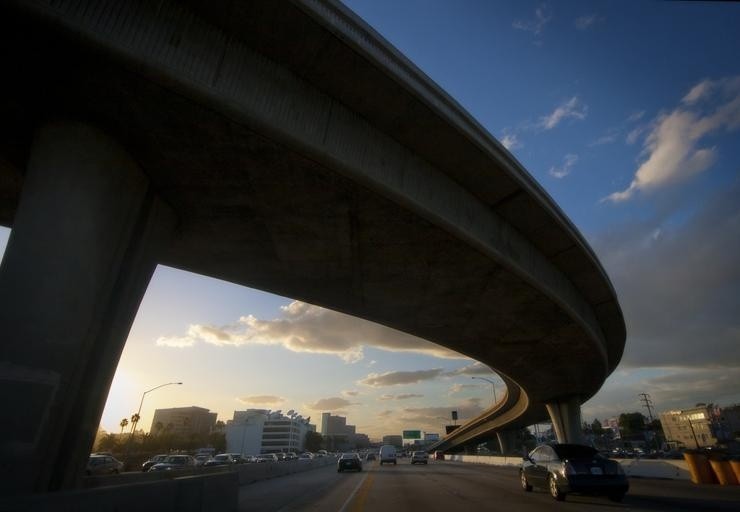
top-left (379, 445), bottom-right (397, 465)
top-left (396, 450), bottom-right (402, 457)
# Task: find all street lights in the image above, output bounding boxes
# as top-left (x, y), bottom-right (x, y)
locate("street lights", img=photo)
top-left (127, 382), bottom-right (183, 454)
top-left (472, 375), bottom-right (497, 405)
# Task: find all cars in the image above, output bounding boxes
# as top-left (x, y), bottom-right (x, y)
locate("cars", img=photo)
top-left (410, 450), bottom-right (445, 465)
top-left (518, 442), bottom-right (630, 502)
top-left (83, 449), bottom-right (336, 476)
top-left (365, 452), bottom-right (376, 462)
top-left (337, 452), bottom-right (363, 473)
